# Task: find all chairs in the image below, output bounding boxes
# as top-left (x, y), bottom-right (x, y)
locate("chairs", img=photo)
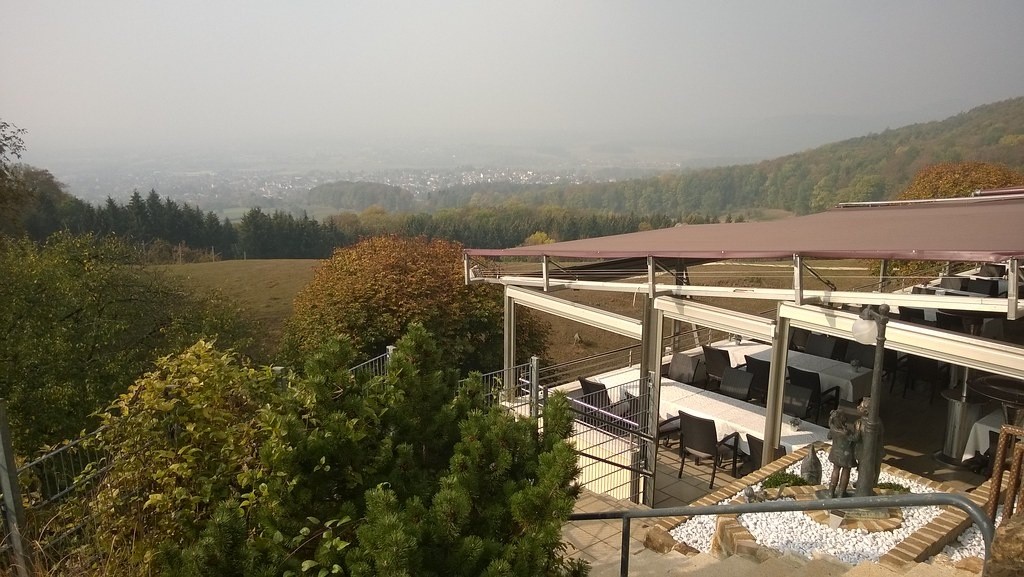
top-left (578, 264), bottom-right (1024, 489)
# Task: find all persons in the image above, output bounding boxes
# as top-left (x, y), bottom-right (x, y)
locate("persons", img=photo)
top-left (821, 396), bottom-right (886, 500)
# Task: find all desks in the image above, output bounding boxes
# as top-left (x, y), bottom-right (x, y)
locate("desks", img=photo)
top-left (961, 408), bottom-right (1024, 473)
top-left (707, 338), bottom-right (887, 407)
top-left (596, 368), bottom-right (836, 457)
top-left (920, 286), bottom-right (992, 298)
